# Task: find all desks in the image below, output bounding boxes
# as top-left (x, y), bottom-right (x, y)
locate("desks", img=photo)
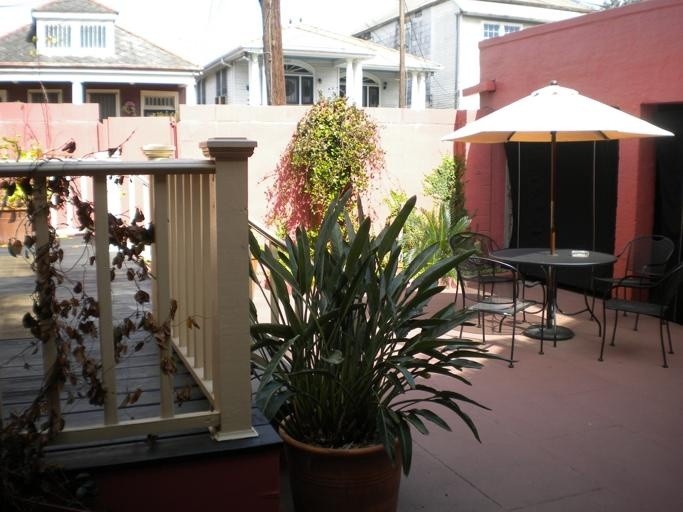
top-left (486, 246), bottom-right (617, 342)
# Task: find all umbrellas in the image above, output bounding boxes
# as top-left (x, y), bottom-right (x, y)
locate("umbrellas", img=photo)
top-left (439, 78), bottom-right (675, 254)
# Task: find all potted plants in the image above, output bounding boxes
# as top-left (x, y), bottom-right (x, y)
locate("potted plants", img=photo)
top-left (247, 180), bottom-right (520, 512)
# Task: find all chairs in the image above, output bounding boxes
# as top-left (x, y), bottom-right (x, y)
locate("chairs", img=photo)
top-left (454, 256), bottom-right (549, 368)
top-left (589, 234), bottom-right (674, 333)
top-left (598, 242), bottom-right (683, 370)
top-left (449, 231), bottom-right (519, 317)
